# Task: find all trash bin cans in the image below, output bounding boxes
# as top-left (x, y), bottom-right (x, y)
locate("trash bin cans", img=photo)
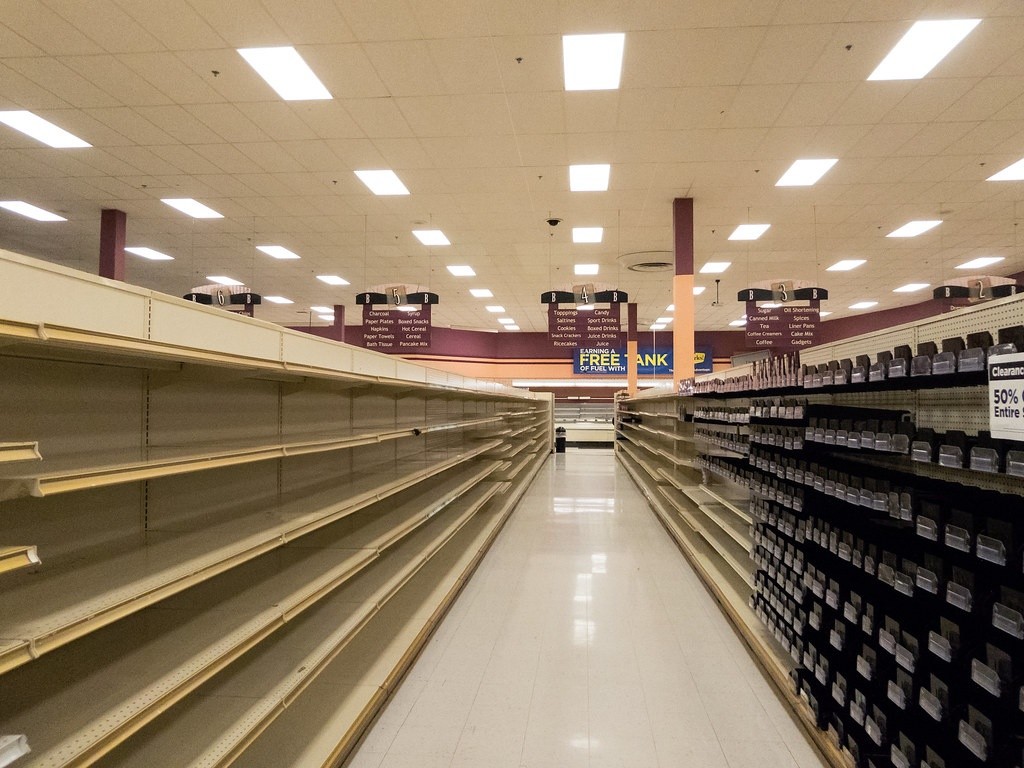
top-left (556, 426), bottom-right (567, 453)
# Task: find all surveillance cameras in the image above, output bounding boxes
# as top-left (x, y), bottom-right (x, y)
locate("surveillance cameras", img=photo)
top-left (546, 219), bottom-right (561, 226)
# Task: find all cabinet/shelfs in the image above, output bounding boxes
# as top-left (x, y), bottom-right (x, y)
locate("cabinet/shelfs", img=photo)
top-left (0, 249), bottom-right (556, 768)
top-left (614, 290), bottom-right (1024, 768)
